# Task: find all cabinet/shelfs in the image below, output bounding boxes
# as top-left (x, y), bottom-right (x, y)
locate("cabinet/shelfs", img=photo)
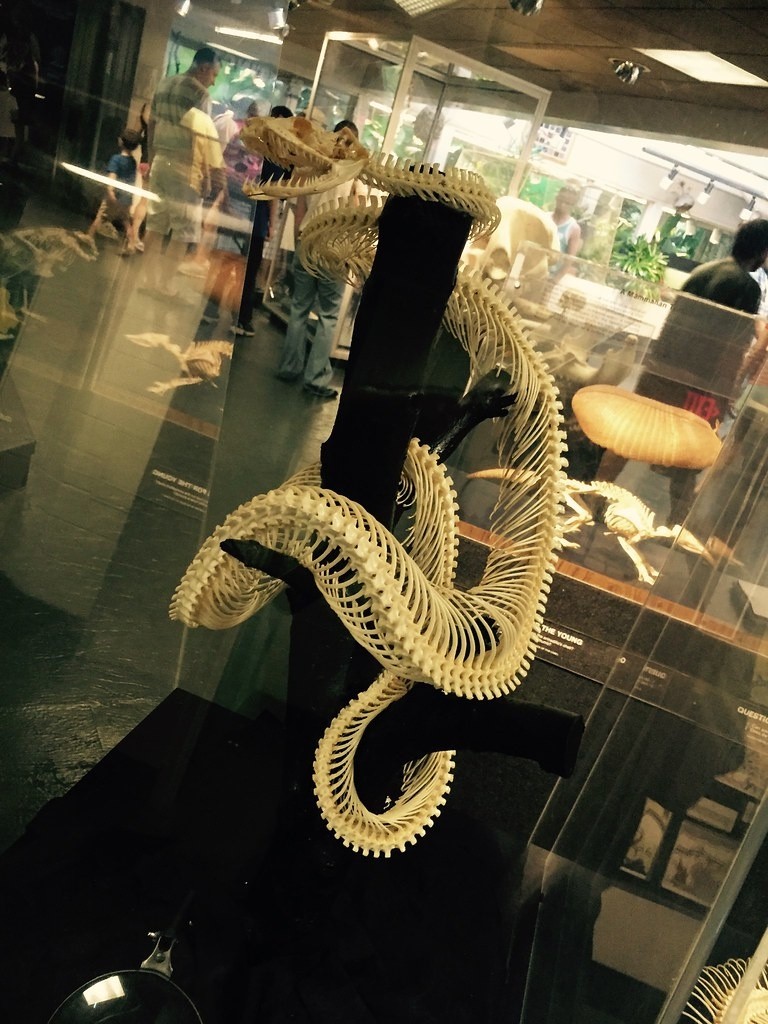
top-left (260, 197), bottom-right (380, 361)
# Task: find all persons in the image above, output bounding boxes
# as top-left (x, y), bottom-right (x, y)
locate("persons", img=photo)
top-left (538, 177), bottom-right (587, 309)
top-left (132, 46), bottom-right (295, 336)
top-left (273, 119), bottom-right (369, 398)
top-left (586, 218), bottom-right (768, 530)
top-left (0, 59), bottom-right (19, 166)
top-left (74, 127), bottom-right (139, 257)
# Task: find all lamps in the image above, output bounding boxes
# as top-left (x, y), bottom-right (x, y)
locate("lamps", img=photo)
top-left (612, 59), bottom-right (640, 85)
top-left (175, 0), bottom-right (190, 17)
top-left (268, 0), bottom-right (300, 30)
top-left (660, 163), bottom-right (757, 220)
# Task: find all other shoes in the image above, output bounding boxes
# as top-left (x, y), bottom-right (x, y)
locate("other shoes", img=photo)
top-left (301, 384), bottom-right (338, 398)
top-left (230, 321), bottom-right (255, 336)
top-left (176, 255), bottom-right (213, 279)
top-left (201, 310), bottom-right (221, 324)
top-left (274, 373), bottom-right (294, 383)
top-left (137, 283), bottom-right (191, 310)
top-left (113, 238), bottom-right (149, 257)
